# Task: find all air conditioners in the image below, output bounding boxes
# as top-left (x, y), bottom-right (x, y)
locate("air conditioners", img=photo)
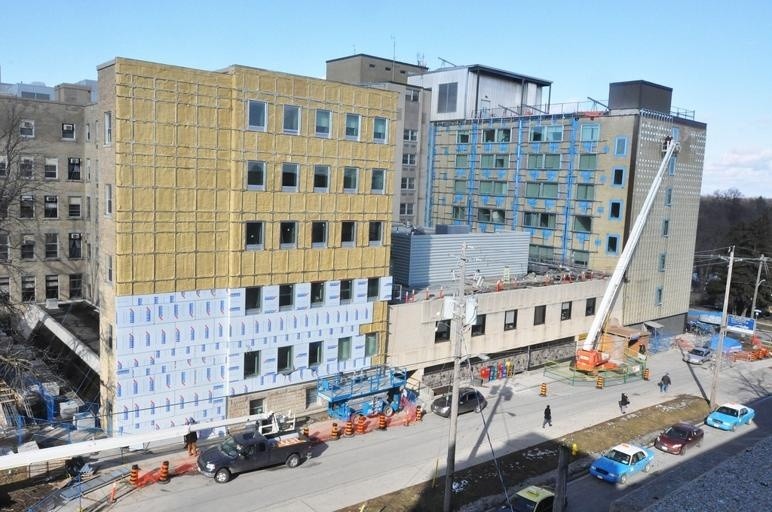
top-left (70, 158), bottom-right (79, 165)
top-left (70, 234), bottom-right (80, 239)
top-left (21, 196), bottom-right (32, 202)
top-left (63, 125), bottom-right (72, 131)
top-left (20, 121), bottom-right (33, 129)
top-left (46, 196), bottom-right (56, 201)
top-left (23, 235), bottom-right (35, 245)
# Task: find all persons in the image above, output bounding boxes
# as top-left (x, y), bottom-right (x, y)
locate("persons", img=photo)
top-left (662, 373), bottom-right (671, 392)
top-left (639, 344), bottom-right (646, 354)
top-left (186, 432), bottom-right (198, 457)
top-left (542, 405), bottom-right (552, 428)
top-left (665, 135), bottom-right (670, 149)
top-left (622, 393), bottom-right (630, 414)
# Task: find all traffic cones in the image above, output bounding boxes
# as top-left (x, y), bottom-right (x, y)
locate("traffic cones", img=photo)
top-left (540, 382), bottom-right (547, 397)
top-left (596, 376), bottom-right (603, 389)
top-left (643, 368), bottom-right (650, 381)
top-left (414, 405), bottom-right (424, 421)
top-left (377, 413), bottom-right (389, 431)
top-left (156, 460), bottom-right (171, 484)
top-left (331, 423), bottom-right (341, 440)
top-left (127, 464), bottom-right (139, 488)
top-left (304, 427), bottom-right (309, 437)
top-left (356, 416), bottom-right (366, 435)
top-left (343, 421), bottom-right (355, 439)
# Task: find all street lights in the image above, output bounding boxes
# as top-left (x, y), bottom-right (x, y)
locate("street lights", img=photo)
top-left (750, 278), bottom-right (766, 320)
top-left (444, 352), bottom-right (490, 510)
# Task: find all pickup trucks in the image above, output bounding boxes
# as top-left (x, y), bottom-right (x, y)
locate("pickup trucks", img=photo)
top-left (195, 423), bottom-right (313, 484)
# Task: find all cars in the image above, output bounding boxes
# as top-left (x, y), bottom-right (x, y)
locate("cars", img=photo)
top-left (431, 386), bottom-right (488, 417)
top-left (587, 441), bottom-right (654, 484)
top-left (704, 401), bottom-right (756, 430)
top-left (501, 484), bottom-right (570, 512)
top-left (651, 422), bottom-right (703, 458)
top-left (683, 346), bottom-right (712, 365)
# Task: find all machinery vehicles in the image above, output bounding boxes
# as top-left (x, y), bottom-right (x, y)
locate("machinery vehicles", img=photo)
top-left (318, 364), bottom-right (417, 424)
top-left (572, 140), bottom-right (684, 377)
